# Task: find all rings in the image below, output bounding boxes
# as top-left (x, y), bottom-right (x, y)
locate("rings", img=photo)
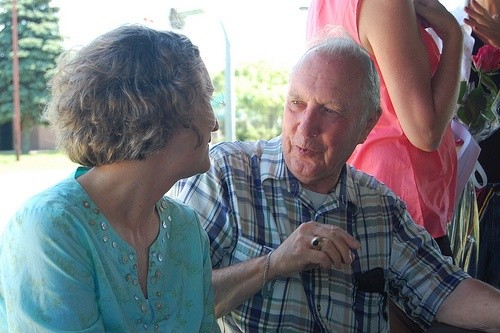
top-left (311, 236), bottom-right (324, 250)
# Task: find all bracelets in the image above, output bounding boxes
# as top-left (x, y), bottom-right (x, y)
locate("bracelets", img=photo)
top-left (260, 250), bottom-right (277, 292)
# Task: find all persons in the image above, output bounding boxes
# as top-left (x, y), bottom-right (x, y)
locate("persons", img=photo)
top-left (0, 23), bottom-right (222, 333)
top-left (305, 0), bottom-right (500, 266)
top-left (155, 24), bottom-right (500, 333)
